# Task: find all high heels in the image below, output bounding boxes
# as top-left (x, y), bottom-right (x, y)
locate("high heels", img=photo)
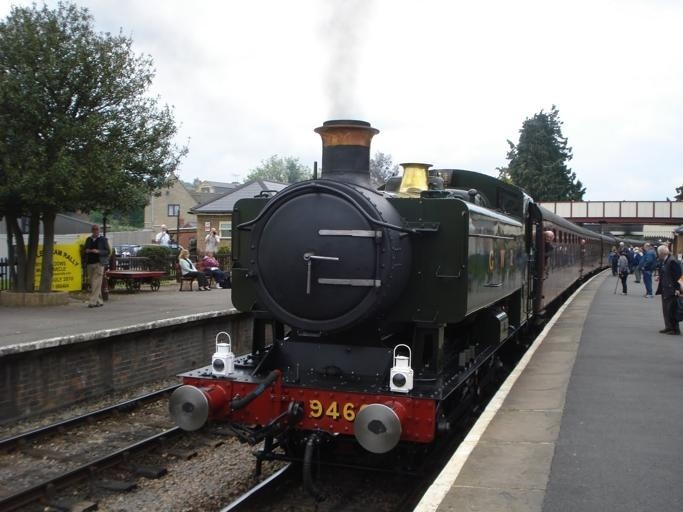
top-left (199, 287), bottom-right (210, 291)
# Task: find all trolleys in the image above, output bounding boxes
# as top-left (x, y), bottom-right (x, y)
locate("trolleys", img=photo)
top-left (107, 270), bottom-right (167, 291)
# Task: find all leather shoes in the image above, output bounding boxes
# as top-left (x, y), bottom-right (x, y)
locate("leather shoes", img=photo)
top-left (658, 328), bottom-right (680, 335)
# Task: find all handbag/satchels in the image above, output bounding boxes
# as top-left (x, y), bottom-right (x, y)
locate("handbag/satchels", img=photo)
top-left (203, 257), bottom-right (219, 267)
top-left (669, 295), bottom-right (682, 323)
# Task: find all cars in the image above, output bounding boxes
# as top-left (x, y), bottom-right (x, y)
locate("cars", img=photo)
top-left (110, 242), bottom-right (184, 259)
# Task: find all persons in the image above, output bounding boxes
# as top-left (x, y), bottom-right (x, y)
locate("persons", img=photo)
top-left (178, 250), bottom-right (211, 291)
top-left (609, 242), bottom-right (660, 298)
top-left (546, 230), bottom-right (554, 253)
top-left (200, 250), bottom-right (225, 289)
top-left (84, 225), bottom-right (111, 308)
top-left (204, 228), bottom-right (220, 255)
top-left (581, 239), bottom-right (586, 251)
top-left (655, 245), bottom-right (681, 335)
top-left (155, 224), bottom-right (171, 250)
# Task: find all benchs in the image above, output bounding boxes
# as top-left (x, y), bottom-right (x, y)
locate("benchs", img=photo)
top-left (175, 264), bottom-right (213, 291)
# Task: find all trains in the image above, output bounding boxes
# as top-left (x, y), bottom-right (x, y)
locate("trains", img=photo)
top-left (167, 120), bottom-right (667, 481)
